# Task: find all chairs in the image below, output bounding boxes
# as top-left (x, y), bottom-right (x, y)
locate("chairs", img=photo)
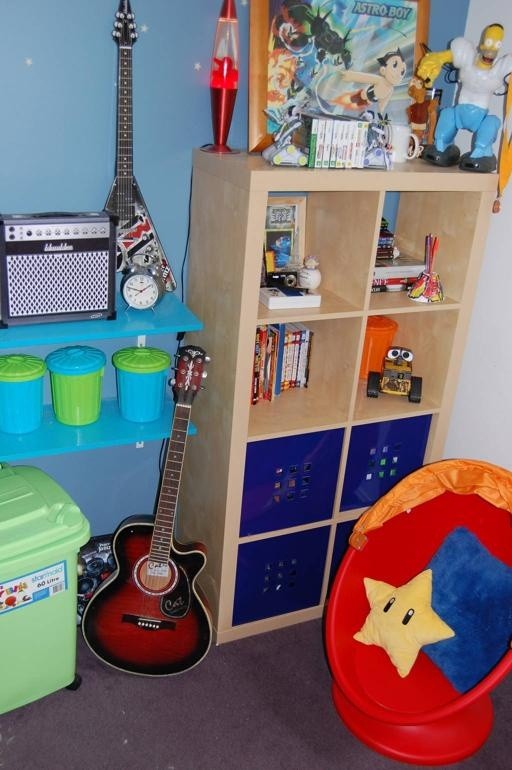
top-left (325, 458), bottom-right (512, 766)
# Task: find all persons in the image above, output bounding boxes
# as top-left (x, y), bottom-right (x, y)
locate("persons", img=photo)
top-left (415, 23), bottom-right (512, 174)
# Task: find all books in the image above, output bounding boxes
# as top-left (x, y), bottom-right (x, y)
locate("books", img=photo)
top-left (371, 254), bottom-right (427, 292)
top-left (264, 0), bottom-right (418, 137)
top-left (292, 112), bottom-right (369, 169)
top-left (252, 322), bottom-right (314, 404)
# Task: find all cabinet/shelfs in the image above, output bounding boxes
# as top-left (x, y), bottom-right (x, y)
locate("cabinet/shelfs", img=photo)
top-left (178, 146), bottom-right (502, 647)
top-left (0, 290), bottom-right (203, 465)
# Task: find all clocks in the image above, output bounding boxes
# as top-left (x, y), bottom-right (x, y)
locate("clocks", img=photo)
top-left (120, 253), bottom-right (167, 315)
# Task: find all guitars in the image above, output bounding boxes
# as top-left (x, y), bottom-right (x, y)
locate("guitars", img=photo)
top-left (82, 345), bottom-right (214, 677)
top-left (103, 0), bottom-right (177, 292)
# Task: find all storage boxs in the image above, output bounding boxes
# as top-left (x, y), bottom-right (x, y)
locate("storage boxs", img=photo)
top-left (0, 463), bottom-right (91, 718)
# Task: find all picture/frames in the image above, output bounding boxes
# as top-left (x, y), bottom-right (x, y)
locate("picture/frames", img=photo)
top-left (262, 196), bottom-right (308, 272)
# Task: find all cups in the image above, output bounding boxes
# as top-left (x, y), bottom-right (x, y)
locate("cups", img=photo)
top-left (387, 123), bottom-right (417, 166)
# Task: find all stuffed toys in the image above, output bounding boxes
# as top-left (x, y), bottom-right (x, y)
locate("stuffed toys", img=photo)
top-left (405, 74), bottom-right (438, 148)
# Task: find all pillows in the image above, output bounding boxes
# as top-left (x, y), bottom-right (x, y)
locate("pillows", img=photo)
top-left (418, 525), bottom-right (512, 695)
